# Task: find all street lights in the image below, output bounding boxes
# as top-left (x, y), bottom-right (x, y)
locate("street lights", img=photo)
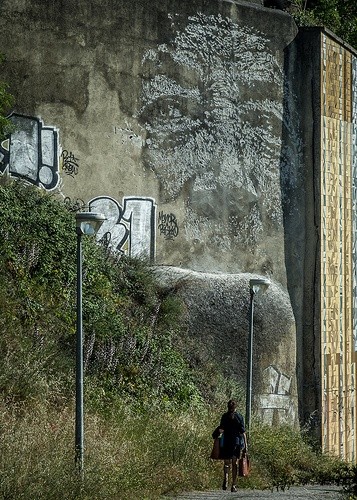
top-left (245, 278), bottom-right (271, 442)
top-left (73, 212), bottom-right (106, 493)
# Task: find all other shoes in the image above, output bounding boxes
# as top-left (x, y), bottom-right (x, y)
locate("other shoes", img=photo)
top-left (231, 484), bottom-right (238, 492)
top-left (222, 481), bottom-right (228, 490)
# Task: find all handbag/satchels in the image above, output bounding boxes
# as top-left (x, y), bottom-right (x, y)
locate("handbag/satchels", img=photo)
top-left (210, 437), bottom-right (221, 460)
top-left (238, 452), bottom-right (249, 477)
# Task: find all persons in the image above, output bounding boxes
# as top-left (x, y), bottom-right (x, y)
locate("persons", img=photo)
top-left (220, 400), bottom-right (248, 493)
